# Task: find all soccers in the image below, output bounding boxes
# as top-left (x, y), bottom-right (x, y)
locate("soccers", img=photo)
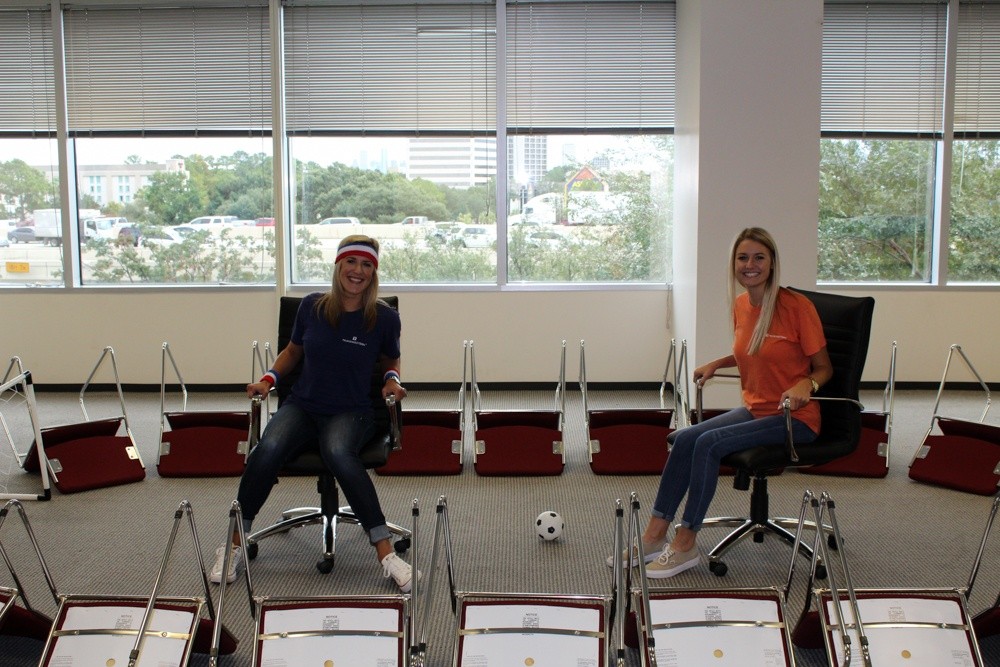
top-left (535, 510), bottom-right (566, 541)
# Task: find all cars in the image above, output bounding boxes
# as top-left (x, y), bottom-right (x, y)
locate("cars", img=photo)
top-left (317, 216), bottom-right (361, 225)
top-left (82, 214), bottom-right (275, 253)
top-left (392, 211), bottom-right (569, 251)
top-left (6, 225), bottom-right (44, 244)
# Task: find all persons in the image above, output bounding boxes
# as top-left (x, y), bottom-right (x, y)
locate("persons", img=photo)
top-left (208, 235), bottom-right (422, 592)
top-left (605, 227), bottom-right (832, 578)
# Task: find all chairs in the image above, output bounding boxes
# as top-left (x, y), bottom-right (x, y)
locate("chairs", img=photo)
top-left (671, 287), bottom-right (875, 580)
top-left (0, 335), bottom-right (1000, 667)
top-left (247, 296), bottom-right (412, 574)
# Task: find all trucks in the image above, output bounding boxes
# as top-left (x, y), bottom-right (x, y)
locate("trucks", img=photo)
top-left (33, 208), bottom-right (103, 248)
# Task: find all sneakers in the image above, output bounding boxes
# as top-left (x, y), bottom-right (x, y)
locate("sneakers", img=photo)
top-left (645, 544), bottom-right (700, 577)
top-left (606, 536), bottom-right (663, 567)
top-left (211, 541), bottom-right (242, 583)
top-left (381, 552), bottom-right (422, 592)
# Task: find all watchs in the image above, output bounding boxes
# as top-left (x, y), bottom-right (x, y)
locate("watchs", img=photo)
top-left (807, 376), bottom-right (819, 394)
top-left (388, 375), bottom-right (401, 385)
